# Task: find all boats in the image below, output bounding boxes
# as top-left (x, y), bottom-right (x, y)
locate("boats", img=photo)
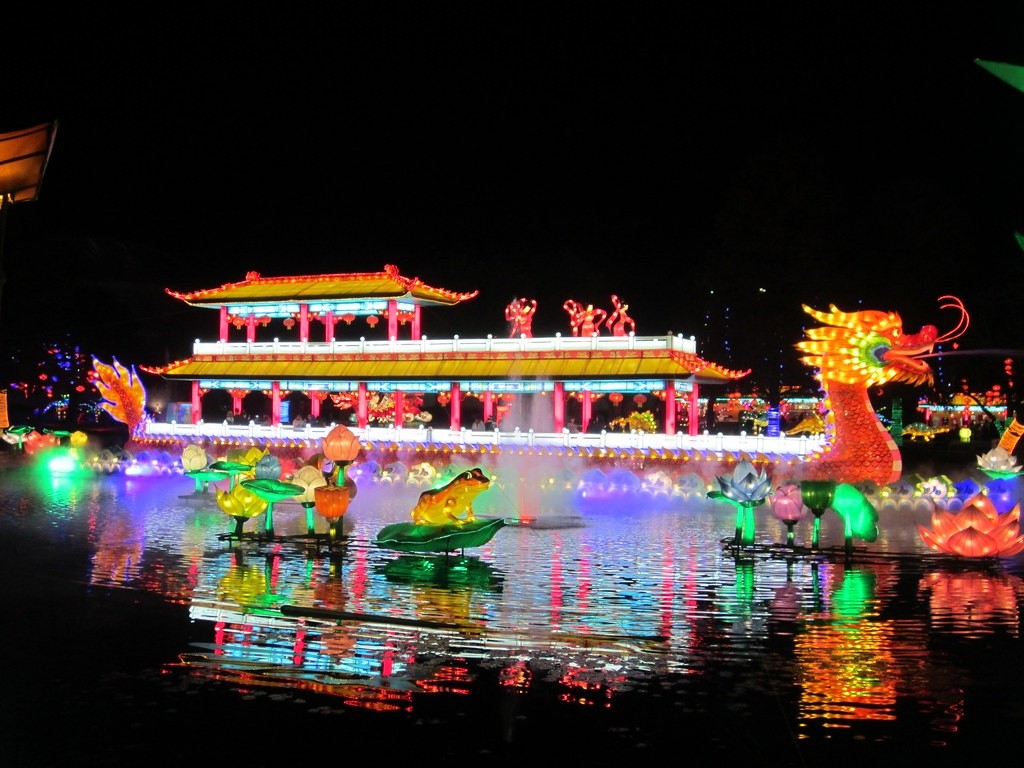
top-left (73, 264), bottom-right (969, 499)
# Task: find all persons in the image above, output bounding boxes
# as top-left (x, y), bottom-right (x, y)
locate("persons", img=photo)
top-left (587, 415), bottom-right (608, 433)
top-left (566, 417), bottom-right (580, 434)
top-left (472, 416), bottom-right (498, 431)
top-left (293, 415), bottom-right (325, 427)
top-left (328, 414), bottom-right (351, 426)
top-left (222, 408), bottom-right (271, 426)
